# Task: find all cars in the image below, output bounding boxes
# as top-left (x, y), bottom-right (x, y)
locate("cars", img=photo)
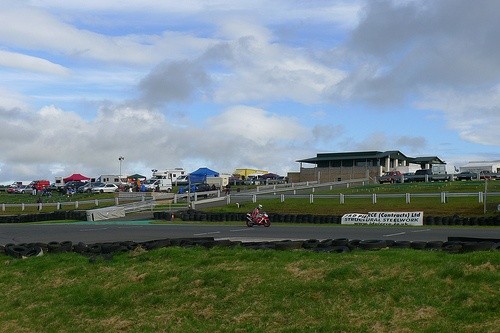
top-left (456, 170), bottom-right (500, 182)
top-left (377, 170), bottom-right (433, 185)
top-left (6, 184), bottom-right (34, 195)
top-left (56, 181), bottom-right (119, 194)
top-left (434, 175), bottom-right (451, 182)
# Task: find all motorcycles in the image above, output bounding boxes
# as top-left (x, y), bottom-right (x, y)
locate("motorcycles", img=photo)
top-left (246, 213), bottom-right (271, 228)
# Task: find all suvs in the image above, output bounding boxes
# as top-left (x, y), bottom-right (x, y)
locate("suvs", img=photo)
top-left (179, 183), bottom-right (212, 201)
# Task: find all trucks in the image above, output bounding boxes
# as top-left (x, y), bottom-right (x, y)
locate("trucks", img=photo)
top-left (54, 177), bottom-right (68, 188)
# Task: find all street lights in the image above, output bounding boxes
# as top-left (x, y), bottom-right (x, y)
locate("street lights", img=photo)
top-left (118, 155), bottom-right (124, 192)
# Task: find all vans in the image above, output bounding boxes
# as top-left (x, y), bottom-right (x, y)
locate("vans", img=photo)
top-left (141, 179), bottom-right (172, 194)
top-left (175, 175), bottom-right (189, 186)
top-left (26, 180), bottom-right (52, 191)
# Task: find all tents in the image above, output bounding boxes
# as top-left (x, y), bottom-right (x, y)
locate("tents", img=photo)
top-left (127, 174), bottom-right (146, 180)
top-left (187, 167), bottom-right (219, 183)
top-left (63, 174), bottom-right (90, 181)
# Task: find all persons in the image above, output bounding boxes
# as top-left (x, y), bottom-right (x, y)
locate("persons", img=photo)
top-left (225, 183), bottom-right (231, 195)
top-left (142, 185), bottom-right (146, 191)
top-left (129, 186), bottom-right (132, 192)
top-left (154, 184), bottom-right (160, 192)
top-left (31, 186), bottom-right (36, 195)
top-left (179, 186), bottom-right (183, 199)
top-left (36, 197), bottom-right (42, 210)
top-left (192, 185), bottom-right (195, 198)
top-left (252, 204), bottom-right (262, 223)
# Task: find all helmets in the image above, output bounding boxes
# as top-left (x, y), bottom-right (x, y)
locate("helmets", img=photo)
top-left (257, 204), bottom-right (263, 209)
